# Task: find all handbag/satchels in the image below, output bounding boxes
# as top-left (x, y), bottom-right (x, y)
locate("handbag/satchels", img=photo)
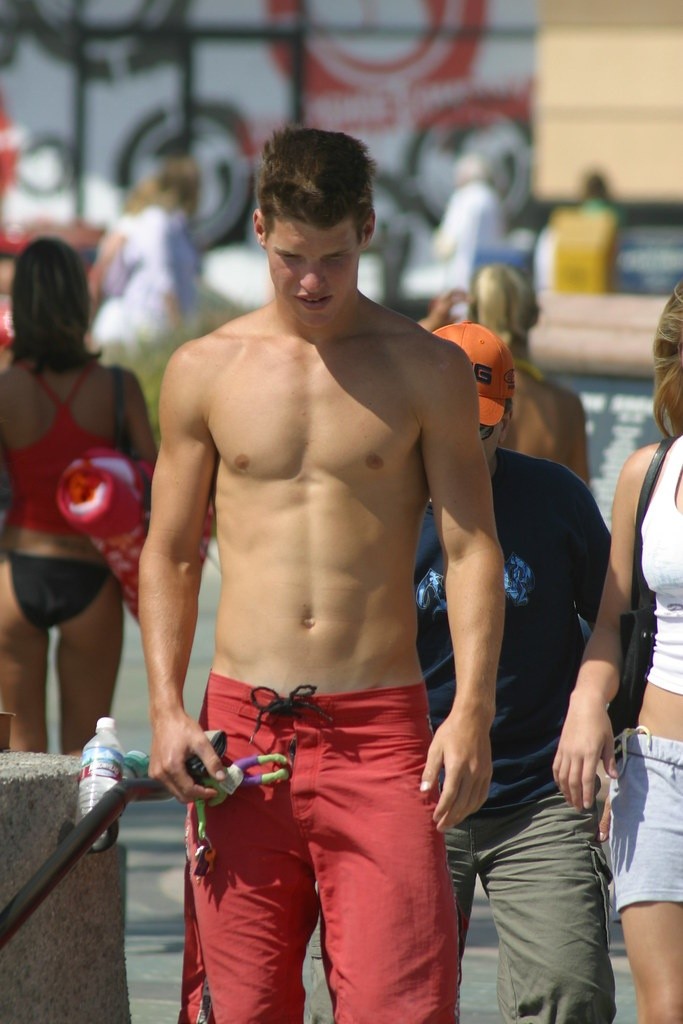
top-left (607, 437), bottom-right (676, 755)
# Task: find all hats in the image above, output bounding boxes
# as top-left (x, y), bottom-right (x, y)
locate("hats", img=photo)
top-left (432, 320), bottom-right (515, 425)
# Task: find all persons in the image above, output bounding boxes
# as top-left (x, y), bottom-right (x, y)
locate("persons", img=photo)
top-left (413, 323), bottom-right (613, 1024)
top-left (552, 286), bottom-right (683, 1024)
top-left (140, 129), bottom-right (507, 1024)
top-left (547, 173), bottom-right (621, 296)
top-left (413, 265), bottom-right (591, 486)
top-left (0, 237), bottom-right (153, 755)
top-left (432, 154), bottom-right (496, 315)
top-left (88, 160), bottom-right (198, 346)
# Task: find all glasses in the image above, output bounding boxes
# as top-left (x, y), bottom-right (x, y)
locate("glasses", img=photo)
top-left (480, 424), bottom-right (496, 441)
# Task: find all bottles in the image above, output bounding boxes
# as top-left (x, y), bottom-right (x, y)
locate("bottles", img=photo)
top-left (123, 750), bottom-right (148, 781)
top-left (74, 717), bottom-right (123, 849)
